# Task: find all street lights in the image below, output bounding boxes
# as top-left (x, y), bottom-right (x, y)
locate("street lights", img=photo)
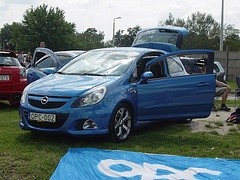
top-left (112, 16), bottom-right (121, 47)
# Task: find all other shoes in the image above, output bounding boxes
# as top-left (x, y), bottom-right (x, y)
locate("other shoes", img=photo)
top-left (220, 105), bottom-right (230, 111)
top-left (211, 104), bottom-right (217, 111)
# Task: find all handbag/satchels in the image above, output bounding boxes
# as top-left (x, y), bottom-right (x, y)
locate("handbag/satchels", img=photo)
top-left (227, 112), bottom-right (240, 123)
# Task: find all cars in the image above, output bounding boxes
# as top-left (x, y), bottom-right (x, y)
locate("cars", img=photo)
top-left (198, 60), bottom-right (226, 81)
top-left (26, 51), bottom-right (86, 75)
top-left (0, 51), bottom-right (27, 105)
top-left (19, 25), bottom-right (216, 142)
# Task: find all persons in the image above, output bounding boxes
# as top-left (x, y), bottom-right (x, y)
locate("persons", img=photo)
top-left (34, 41), bottom-right (47, 62)
top-left (202, 66), bottom-right (232, 111)
top-left (25, 52), bottom-right (32, 63)
top-left (16, 51), bottom-right (24, 63)
